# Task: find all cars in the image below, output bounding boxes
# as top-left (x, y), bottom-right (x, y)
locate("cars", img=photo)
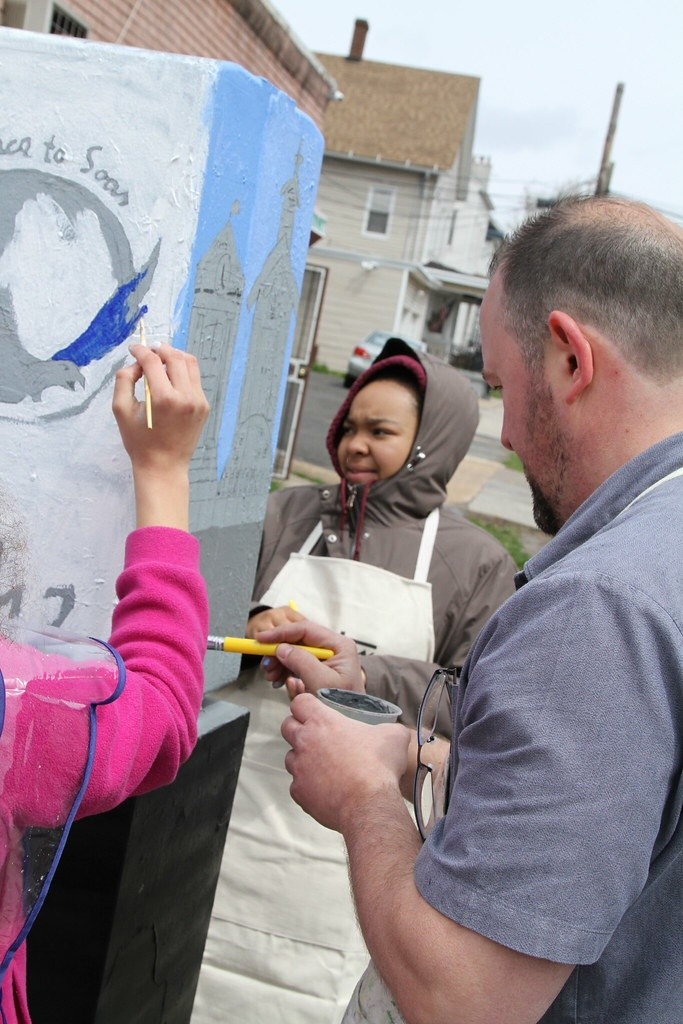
top-left (344, 329), bottom-right (428, 395)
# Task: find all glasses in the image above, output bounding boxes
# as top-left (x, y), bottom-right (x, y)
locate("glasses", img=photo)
top-left (414, 665), bottom-right (460, 844)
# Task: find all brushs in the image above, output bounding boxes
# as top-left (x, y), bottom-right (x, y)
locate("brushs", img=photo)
top-left (207, 633), bottom-right (336, 662)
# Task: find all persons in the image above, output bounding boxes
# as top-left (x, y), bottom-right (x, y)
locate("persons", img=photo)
top-left (0, 342), bottom-right (210, 1024)
top-left (247, 335), bottom-right (519, 738)
top-left (255, 196), bottom-right (683, 1024)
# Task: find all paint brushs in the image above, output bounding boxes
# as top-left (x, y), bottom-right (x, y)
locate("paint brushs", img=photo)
top-left (135, 302), bottom-right (154, 431)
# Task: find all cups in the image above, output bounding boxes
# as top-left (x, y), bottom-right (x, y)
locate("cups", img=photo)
top-left (317, 687), bottom-right (403, 724)
